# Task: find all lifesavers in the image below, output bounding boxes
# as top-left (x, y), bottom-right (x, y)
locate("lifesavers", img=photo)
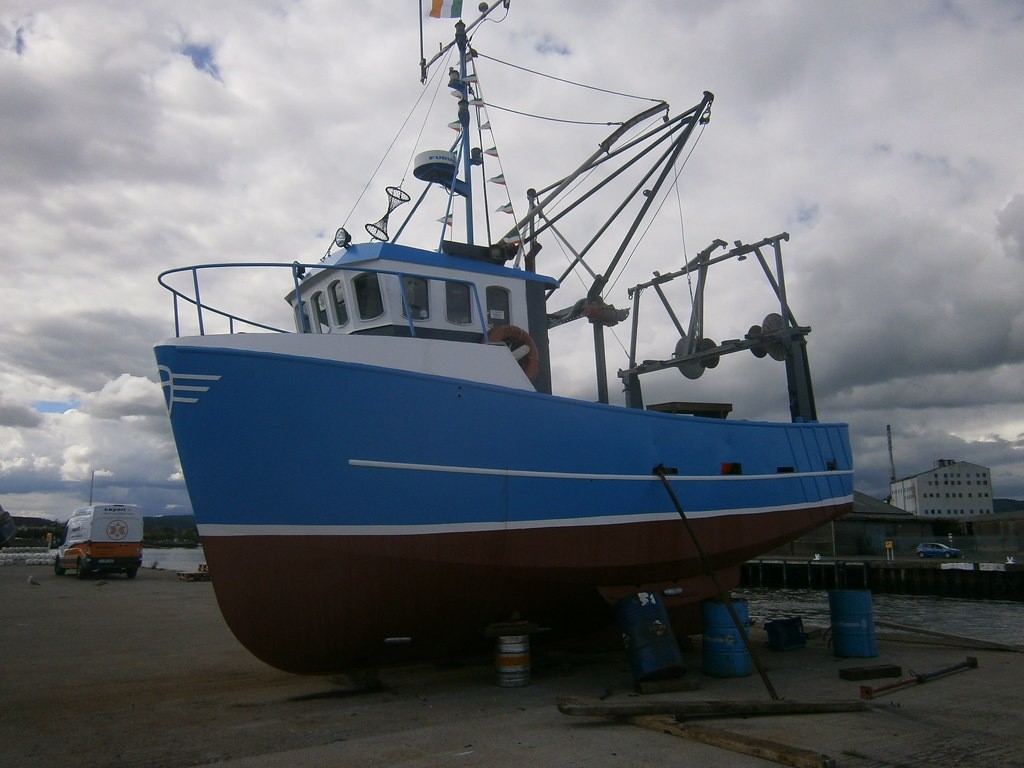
top-left (481, 324), bottom-right (541, 385)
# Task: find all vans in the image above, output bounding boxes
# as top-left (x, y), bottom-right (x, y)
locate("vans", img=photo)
top-left (54, 503), bottom-right (144, 579)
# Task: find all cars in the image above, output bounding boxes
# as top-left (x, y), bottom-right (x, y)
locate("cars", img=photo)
top-left (916, 542), bottom-right (961, 558)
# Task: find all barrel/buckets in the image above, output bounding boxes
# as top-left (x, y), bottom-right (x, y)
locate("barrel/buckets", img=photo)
top-left (494, 632), bottom-right (531, 688)
top-left (703, 596), bottom-right (750, 678)
top-left (763, 613), bottom-right (808, 651)
top-left (828, 589), bottom-right (878, 658)
top-left (616, 590), bottom-right (684, 680)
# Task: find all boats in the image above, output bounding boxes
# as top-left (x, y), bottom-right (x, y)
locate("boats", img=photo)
top-left (158, 538), bottom-right (198, 548)
top-left (152, 0), bottom-right (855, 673)
